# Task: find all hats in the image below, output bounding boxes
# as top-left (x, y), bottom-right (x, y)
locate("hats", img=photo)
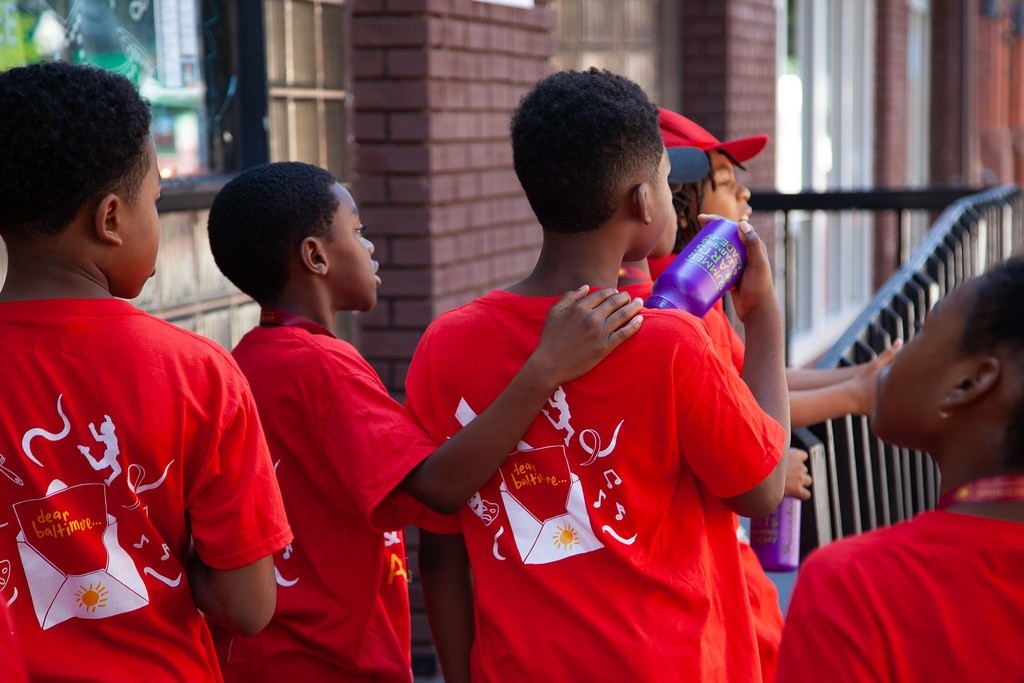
top-left (656, 104), bottom-right (768, 167)
top-left (665, 147), bottom-right (710, 184)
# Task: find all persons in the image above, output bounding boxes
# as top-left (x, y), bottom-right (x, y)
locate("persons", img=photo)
top-left (618, 107), bottom-right (903, 500)
top-left (775, 256), bottom-right (1024, 683)
top-left (207, 161), bottom-right (643, 683)
top-left (403, 69), bottom-right (792, 683)
top-left (0, 62), bottom-right (294, 683)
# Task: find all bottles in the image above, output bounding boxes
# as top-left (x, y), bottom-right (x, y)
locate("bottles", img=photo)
top-left (749, 496), bottom-right (801, 571)
top-left (642, 218), bottom-right (746, 317)
